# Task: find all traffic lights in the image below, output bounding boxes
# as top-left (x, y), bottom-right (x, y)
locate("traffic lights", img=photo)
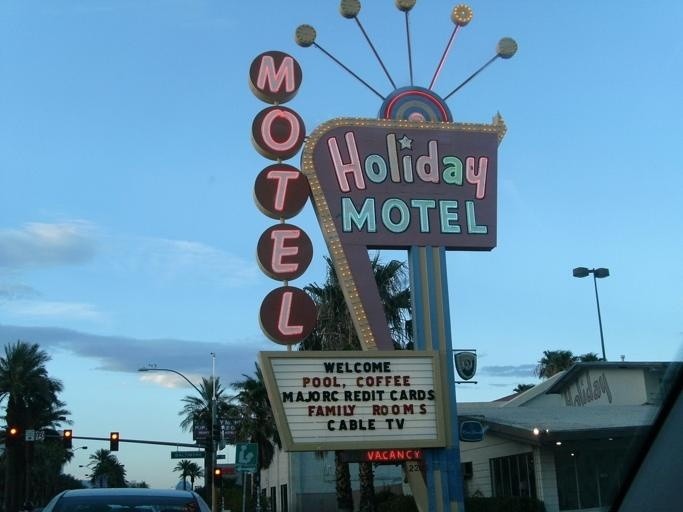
top-left (62, 429), bottom-right (71, 448)
top-left (213, 467), bottom-right (222, 489)
top-left (109, 431), bottom-right (118, 451)
top-left (7, 426), bottom-right (17, 445)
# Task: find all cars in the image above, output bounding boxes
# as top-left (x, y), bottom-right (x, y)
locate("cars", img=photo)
top-left (31, 487), bottom-right (211, 512)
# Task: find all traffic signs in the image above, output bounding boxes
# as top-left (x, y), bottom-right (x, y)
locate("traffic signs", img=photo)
top-left (170, 451), bottom-right (205, 458)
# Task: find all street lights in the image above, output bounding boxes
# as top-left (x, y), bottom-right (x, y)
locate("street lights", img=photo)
top-left (136, 368), bottom-right (217, 511)
top-left (54, 445), bottom-right (87, 495)
top-left (572, 267), bottom-right (610, 362)
top-left (212, 424), bottom-right (220, 442)
top-left (78, 465), bottom-right (104, 488)
top-left (84, 473), bottom-right (97, 487)
